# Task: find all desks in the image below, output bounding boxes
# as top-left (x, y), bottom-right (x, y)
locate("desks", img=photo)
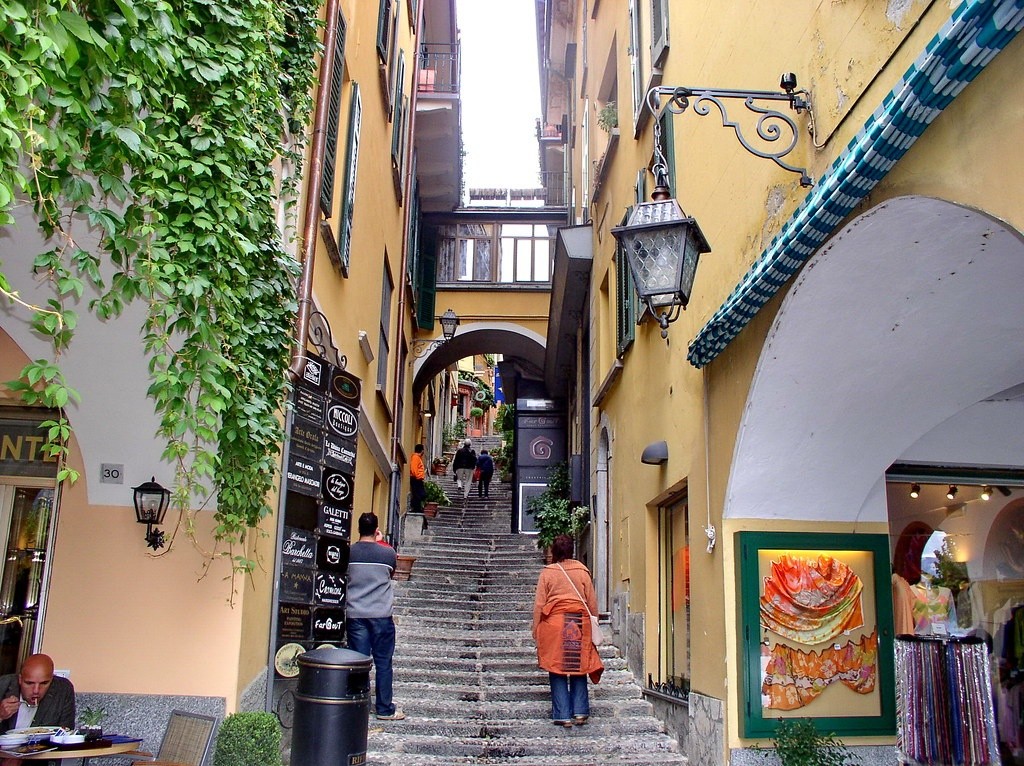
top-left (0, 734), bottom-right (143, 765)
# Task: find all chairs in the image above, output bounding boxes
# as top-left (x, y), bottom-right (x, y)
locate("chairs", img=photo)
top-left (129, 708), bottom-right (219, 766)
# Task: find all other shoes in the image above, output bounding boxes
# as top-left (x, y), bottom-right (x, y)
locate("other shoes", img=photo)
top-left (575, 716), bottom-right (587, 725)
top-left (554, 720), bottom-right (572, 727)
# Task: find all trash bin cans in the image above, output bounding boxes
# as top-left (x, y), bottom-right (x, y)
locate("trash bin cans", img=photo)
top-left (291, 648), bottom-right (374, 766)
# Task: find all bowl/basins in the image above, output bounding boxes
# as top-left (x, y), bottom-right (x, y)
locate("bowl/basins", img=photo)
top-left (0, 734), bottom-right (27, 750)
top-left (5, 728), bottom-right (55, 742)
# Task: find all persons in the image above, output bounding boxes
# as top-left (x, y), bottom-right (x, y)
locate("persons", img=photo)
top-left (910, 574), bottom-right (957, 634)
top-left (410, 444), bottom-right (425, 511)
top-left (477, 450), bottom-right (494, 498)
top-left (0, 653), bottom-right (75, 766)
top-left (533, 538), bottom-right (604, 727)
top-left (347, 512), bottom-right (405, 719)
top-left (453, 439), bottom-right (476, 498)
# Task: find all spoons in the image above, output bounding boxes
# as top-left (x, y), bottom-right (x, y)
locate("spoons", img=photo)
top-left (18, 701), bottom-right (38, 708)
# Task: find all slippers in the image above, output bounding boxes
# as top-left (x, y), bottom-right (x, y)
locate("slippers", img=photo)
top-left (377, 710), bottom-right (405, 720)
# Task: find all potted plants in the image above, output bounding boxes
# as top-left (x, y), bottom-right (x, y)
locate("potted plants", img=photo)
top-left (79, 708), bottom-right (109, 732)
top-left (440, 435), bottom-right (456, 462)
top-left (527, 461), bottom-right (591, 564)
top-left (432, 457), bottom-right (451, 476)
top-left (421, 481), bottom-right (452, 518)
top-left (441, 424), bottom-right (460, 449)
top-left (491, 444), bottom-right (515, 483)
top-left (471, 407), bottom-right (483, 418)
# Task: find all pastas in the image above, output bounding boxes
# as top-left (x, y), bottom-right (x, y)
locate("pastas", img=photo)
top-left (22, 728), bottom-right (51, 734)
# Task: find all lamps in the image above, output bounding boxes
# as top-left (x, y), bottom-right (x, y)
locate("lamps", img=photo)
top-left (945, 484), bottom-right (957, 501)
top-left (610, 75), bottom-right (814, 337)
top-left (982, 483), bottom-right (994, 504)
top-left (408, 309), bottom-right (460, 367)
top-left (909, 483), bottom-right (923, 500)
top-left (130, 475), bottom-right (173, 551)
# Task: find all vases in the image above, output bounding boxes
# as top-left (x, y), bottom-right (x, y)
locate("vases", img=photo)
top-left (391, 555), bottom-right (417, 581)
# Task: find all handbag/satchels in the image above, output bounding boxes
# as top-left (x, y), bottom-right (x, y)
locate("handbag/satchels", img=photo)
top-left (475, 468), bottom-right (480, 481)
top-left (591, 616), bottom-right (604, 646)
top-left (472, 474), bottom-right (476, 483)
